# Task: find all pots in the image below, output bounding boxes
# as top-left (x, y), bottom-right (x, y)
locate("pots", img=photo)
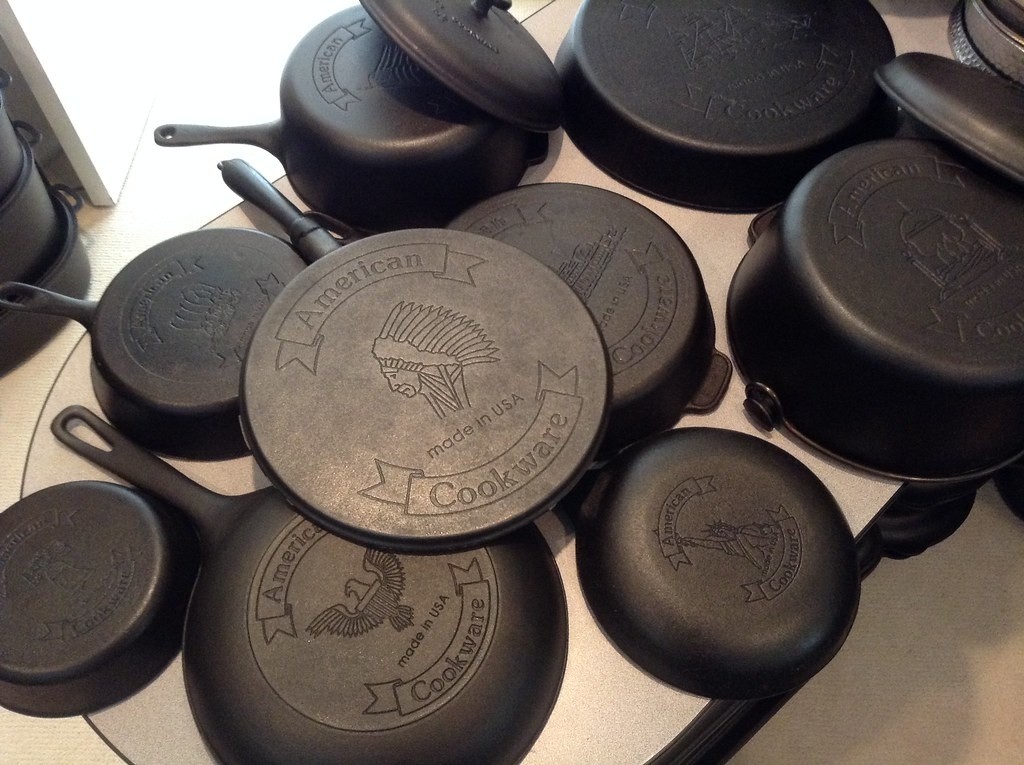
top-left (0, 1), bottom-right (1024, 764)
